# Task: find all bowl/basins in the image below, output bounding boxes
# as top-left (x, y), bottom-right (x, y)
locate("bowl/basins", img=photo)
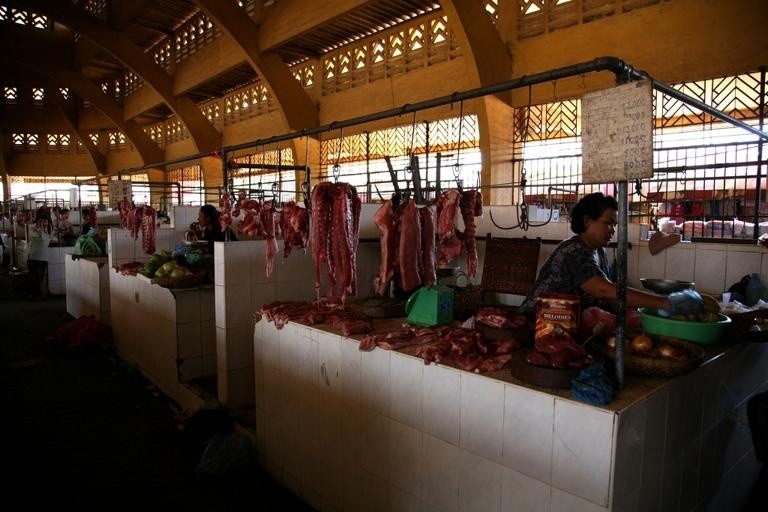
top-left (640, 277), bottom-right (697, 293)
top-left (601, 333), bottom-right (703, 378)
top-left (634, 306), bottom-right (732, 345)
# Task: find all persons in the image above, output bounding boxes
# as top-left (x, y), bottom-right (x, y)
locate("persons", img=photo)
top-left (520, 191), bottom-right (703, 315)
top-left (192, 202), bottom-right (236, 254)
top-left (26, 213), bottom-right (54, 301)
top-left (80, 208), bottom-right (97, 236)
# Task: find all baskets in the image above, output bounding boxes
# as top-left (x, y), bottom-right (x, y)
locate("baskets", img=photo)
top-left (605, 330), bottom-right (706, 376)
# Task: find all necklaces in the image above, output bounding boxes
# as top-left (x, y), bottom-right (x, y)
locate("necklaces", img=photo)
top-left (56, 208), bottom-right (72, 233)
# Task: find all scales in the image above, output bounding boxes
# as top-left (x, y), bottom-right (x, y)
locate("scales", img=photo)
top-left (640, 277), bottom-right (696, 296)
top-left (403, 266), bottom-right (462, 329)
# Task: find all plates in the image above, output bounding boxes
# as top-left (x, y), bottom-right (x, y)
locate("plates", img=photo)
top-left (183, 239), bottom-right (208, 246)
